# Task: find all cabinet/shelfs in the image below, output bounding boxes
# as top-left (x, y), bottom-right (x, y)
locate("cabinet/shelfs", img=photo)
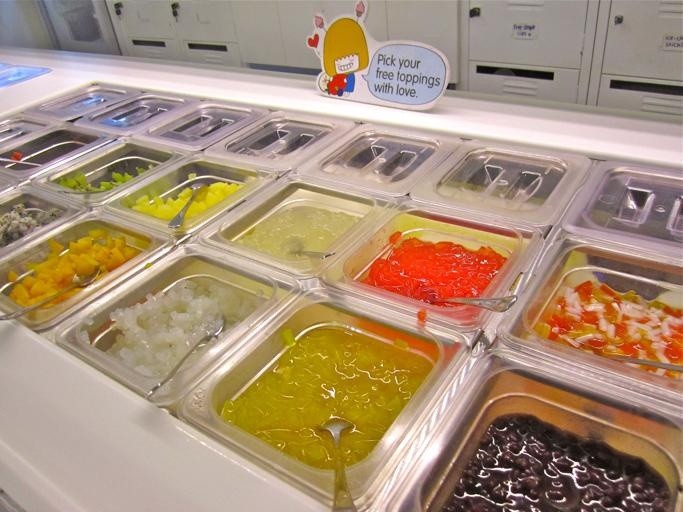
top-left (105, 1), bottom-right (683, 115)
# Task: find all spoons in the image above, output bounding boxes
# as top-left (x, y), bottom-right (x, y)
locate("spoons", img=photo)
top-left (0, 263), bottom-right (102, 323)
top-left (317, 418), bottom-right (360, 511)
top-left (167, 180), bottom-right (210, 231)
top-left (143, 312), bottom-right (227, 402)
